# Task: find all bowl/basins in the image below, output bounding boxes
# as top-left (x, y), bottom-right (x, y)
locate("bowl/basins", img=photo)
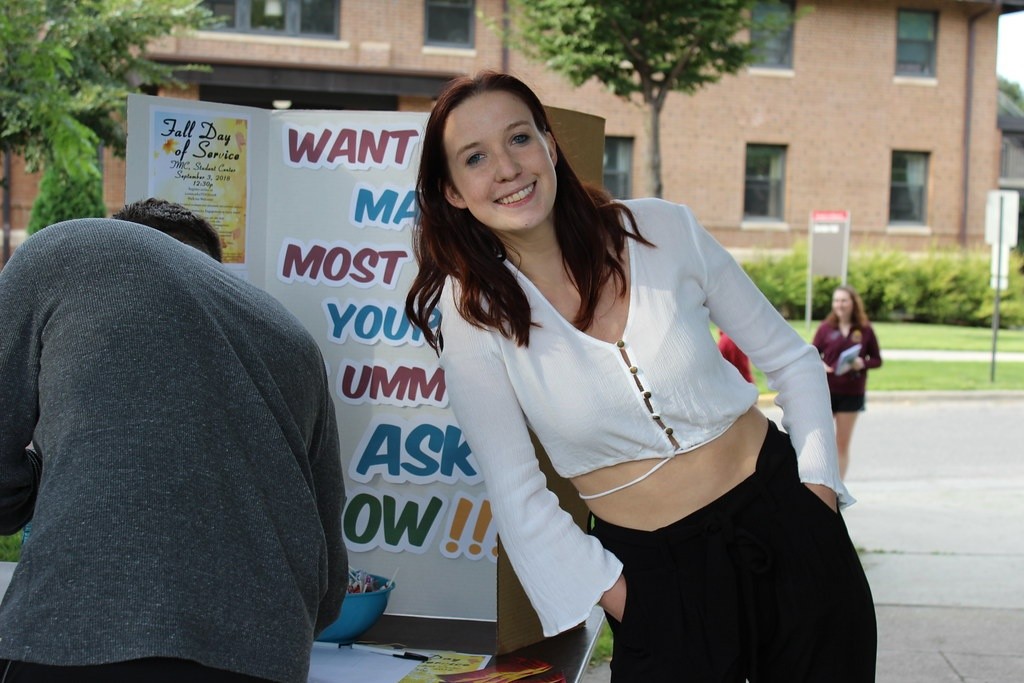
top-left (316, 573), bottom-right (396, 644)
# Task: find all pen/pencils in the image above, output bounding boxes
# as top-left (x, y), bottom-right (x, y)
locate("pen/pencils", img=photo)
top-left (313, 641), bottom-right (342, 649)
top-left (350, 643), bottom-right (430, 662)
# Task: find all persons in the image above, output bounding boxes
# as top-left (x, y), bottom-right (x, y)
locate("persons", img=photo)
top-left (0, 198), bottom-right (349, 683)
top-left (403, 70), bottom-right (877, 683)
top-left (717, 285), bottom-right (882, 483)
